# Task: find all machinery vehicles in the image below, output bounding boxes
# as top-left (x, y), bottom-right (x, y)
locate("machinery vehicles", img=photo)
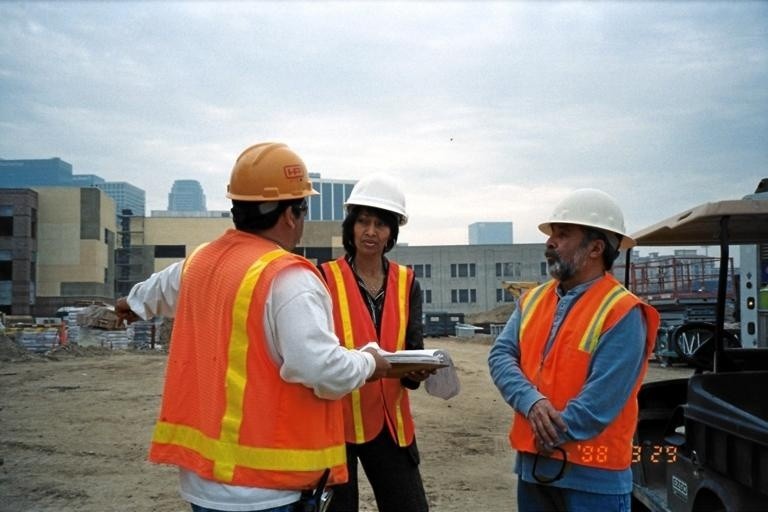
top-left (622, 199), bottom-right (767, 511)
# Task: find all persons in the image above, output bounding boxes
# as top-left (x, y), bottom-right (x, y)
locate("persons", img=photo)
top-left (488, 189), bottom-right (660, 511)
top-left (115, 143), bottom-right (393, 511)
top-left (315, 174), bottom-right (438, 512)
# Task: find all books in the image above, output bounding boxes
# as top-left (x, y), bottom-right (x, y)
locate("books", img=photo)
top-left (359, 342), bottom-right (461, 402)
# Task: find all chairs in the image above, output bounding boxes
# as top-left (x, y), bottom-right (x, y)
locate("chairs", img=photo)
top-left (662, 348), bottom-right (768, 454)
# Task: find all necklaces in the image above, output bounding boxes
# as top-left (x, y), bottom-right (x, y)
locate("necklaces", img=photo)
top-left (353, 263), bottom-right (381, 293)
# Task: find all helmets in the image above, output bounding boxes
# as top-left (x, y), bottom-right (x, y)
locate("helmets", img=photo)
top-left (538, 190), bottom-right (636, 252)
top-left (226, 142), bottom-right (320, 202)
top-left (344, 176), bottom-right (407, 226)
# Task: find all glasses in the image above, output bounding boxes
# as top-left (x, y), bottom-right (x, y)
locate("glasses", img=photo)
top-left (532, 446), bottom-right (572, 484)
